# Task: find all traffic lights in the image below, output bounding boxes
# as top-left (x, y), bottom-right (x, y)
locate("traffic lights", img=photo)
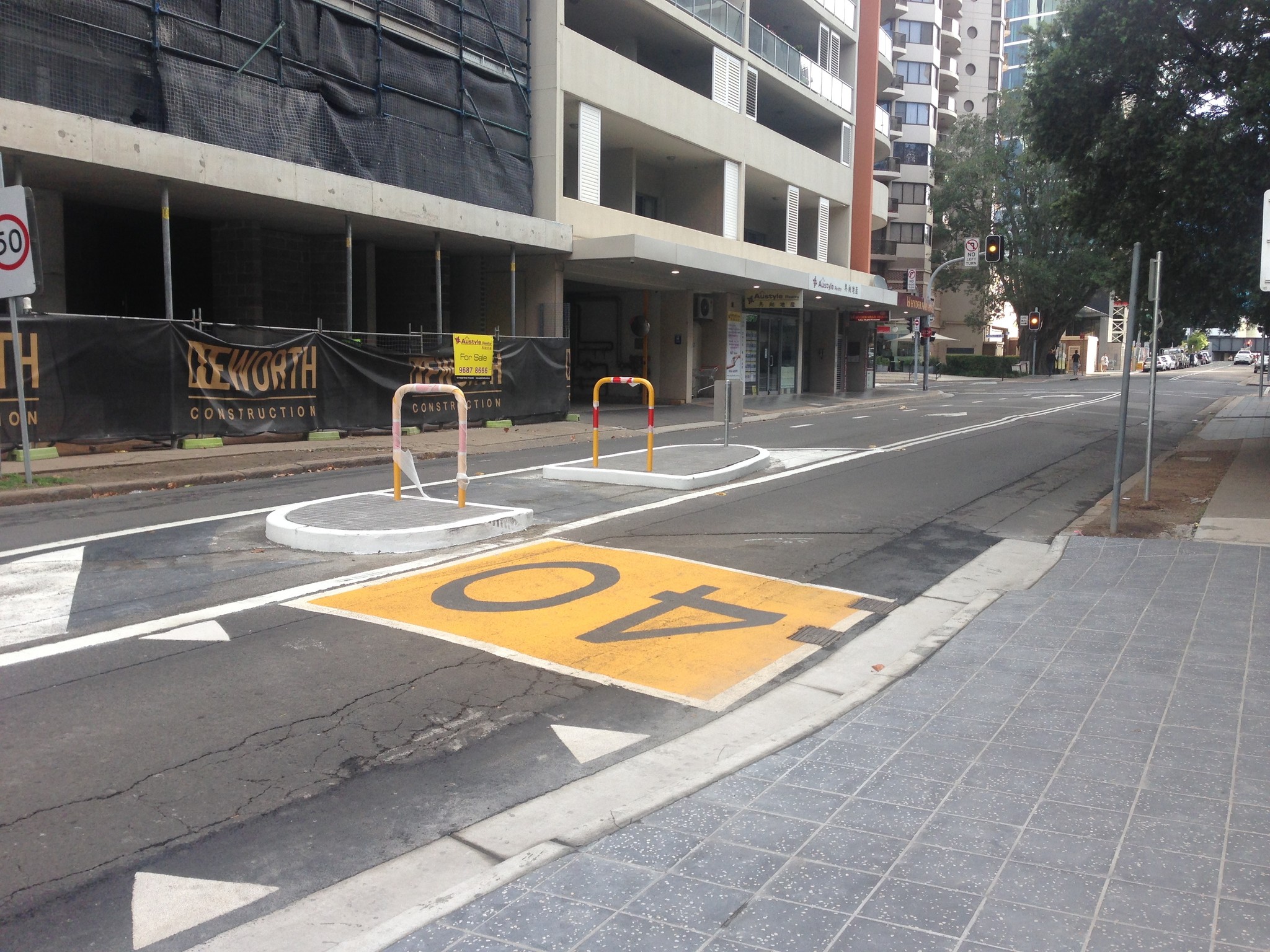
top-left (930, 331), bottom-right (936, 342)
top-left (1247, 342), bottom-right (1250, 346)
top-left (1028, 311), bottom-right (1040, 331)
top-left (903, 269), bottom-right (910, 291)
top-left (906, 317), bottom-right (913, 333)
top-left (985, 235), bottom-right (1001, 263)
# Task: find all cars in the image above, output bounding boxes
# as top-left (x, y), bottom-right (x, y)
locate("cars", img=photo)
top-left (1233, 350), bottom-right (1255, 365)
top-left (1254, 355), bottom-right (1269, 374)
top-left (1142, 346), bottom-right (1211, 372)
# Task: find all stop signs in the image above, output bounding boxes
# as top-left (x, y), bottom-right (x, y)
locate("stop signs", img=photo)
top-left (914, 319), bottom-right (920, 329)
top-left (921, 327), bottom-right (932, 338)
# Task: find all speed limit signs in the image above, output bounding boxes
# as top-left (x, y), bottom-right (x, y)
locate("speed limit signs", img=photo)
top-left (0, 184), bottom-right (38, 299)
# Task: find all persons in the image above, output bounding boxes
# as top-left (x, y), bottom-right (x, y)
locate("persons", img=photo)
top-left (1071, 350), bottom-right (1081, 375)
top-left (1046, 350), bottom-right (1059, 377)
top-left (1101, 353), bottom-right (1109, 372)
top-left (1189, 352), bottom-right (1194, 367)
top-left (1197, 352), bottom-right (1202, 367)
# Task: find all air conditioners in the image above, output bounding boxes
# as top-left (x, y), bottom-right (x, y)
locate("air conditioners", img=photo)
top-left (697, 297), bottom-right (714, 319)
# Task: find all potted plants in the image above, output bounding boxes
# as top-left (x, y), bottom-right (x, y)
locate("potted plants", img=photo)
top-left (903, 356), bottom-right (937, 373)
top-left (875, 356), bottom-right (890, 372)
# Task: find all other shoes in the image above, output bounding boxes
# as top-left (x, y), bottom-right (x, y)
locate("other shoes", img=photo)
top-left (1073, 372), bottom-right (1078, 375)
top-left (1049, 372), bottom-right (1053, 376)
top-left (1103, 370), bottom-right (1106, 372)
